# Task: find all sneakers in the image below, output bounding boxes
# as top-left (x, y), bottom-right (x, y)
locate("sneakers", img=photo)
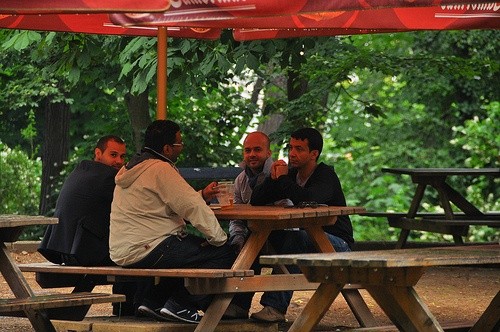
top-left (160, 299), bottom-right (201, 323)
top-left (138, 303), bottom-right (174, 321)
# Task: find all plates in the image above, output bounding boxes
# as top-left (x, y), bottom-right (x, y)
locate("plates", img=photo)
top-left (210, 207), bottom-right (221, 209)
top-left (211, 203), bottom-right (220, 205)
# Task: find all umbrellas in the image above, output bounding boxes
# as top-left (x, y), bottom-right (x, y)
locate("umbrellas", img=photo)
top-left (0, 0), bottom-right (500, 120)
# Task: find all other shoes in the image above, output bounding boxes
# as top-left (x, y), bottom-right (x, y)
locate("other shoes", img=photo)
top-left (250, 306), bottom-right (285, 323)
top-left (221, 304), bottom-right (249, 319)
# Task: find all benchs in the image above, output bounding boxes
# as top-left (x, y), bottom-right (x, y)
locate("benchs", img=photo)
top-left (0, 210), bottom-right (500, 332)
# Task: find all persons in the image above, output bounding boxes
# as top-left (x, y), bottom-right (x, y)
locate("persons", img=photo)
top-left (37, 135), bottom-right (156, 315)
top-left (109, 120), bottom-right (241, 322)
top-left (222, 127), bottom-right (355, 322)
top-left (229, 131), bottom-right (300, 252)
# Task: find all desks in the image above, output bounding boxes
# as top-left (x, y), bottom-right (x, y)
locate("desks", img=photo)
top-left (380, 167), bottom-right (500, 243)
top-left (0, 214), bottom-right (59, 332)
top-left (194, 201), bottom-right (379, 332)
top-left (259, 244), bottom-right (500, 332)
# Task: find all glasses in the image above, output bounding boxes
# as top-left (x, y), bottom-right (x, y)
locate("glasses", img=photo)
top-left (172, 141), bottom-right (184, 147)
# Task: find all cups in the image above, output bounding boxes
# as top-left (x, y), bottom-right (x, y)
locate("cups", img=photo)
top-left (212, 181), bottom-right (235, 210)
